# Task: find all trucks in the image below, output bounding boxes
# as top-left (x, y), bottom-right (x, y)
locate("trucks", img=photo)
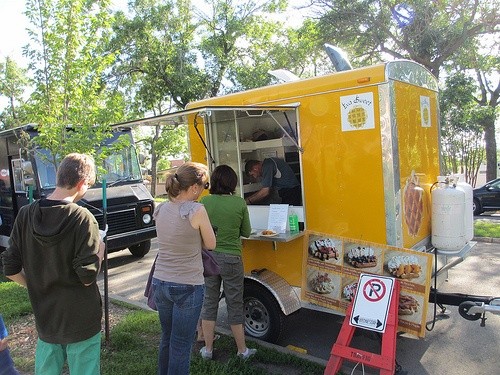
top-left (0, 123), bottom-right (157, 258)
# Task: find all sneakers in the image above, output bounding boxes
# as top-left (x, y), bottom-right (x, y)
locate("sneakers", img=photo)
top-left (200, 346), bottom-right (212, 361)
top-left (236, 348), bottom-right (257, 360)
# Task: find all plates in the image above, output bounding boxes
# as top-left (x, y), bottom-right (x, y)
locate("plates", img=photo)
top-left (257, 231), bottom-right (278, 237)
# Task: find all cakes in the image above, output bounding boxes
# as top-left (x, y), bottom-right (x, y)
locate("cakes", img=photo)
top-left (310, 237), bottom-right (421, 314)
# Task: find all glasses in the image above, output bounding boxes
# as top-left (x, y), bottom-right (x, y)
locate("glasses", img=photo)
top-left (203, 182), bottom-right (209, 189)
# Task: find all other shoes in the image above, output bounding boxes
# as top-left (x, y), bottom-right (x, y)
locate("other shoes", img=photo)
top-left (197, 335), bottom-right (220, 342)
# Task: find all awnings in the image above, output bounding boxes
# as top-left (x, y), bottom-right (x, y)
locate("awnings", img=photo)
top-left (110, 104), bottom-right (297, 128)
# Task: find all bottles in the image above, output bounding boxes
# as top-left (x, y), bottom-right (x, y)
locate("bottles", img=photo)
top-left (289, 205), bottom-right (299, 233)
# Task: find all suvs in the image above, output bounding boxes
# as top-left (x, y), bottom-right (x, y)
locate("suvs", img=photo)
top-left (471, 178), bottom-right (500, 217)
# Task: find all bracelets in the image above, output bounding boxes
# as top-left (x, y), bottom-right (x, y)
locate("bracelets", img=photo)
top-left (247, 197), bottom-right (252, 205)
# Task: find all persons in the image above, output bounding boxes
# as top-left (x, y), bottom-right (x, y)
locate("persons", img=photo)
top-left (151, 164), bottom-right (216, 375)
top-left (2, 153), bottom-right (105, 375)
top-left (198, 165), bottom-right (257, 360)
top-left (0, 314), bottom-right (20, 375)
top-left (244, 158), bottom-right (302, 206)
